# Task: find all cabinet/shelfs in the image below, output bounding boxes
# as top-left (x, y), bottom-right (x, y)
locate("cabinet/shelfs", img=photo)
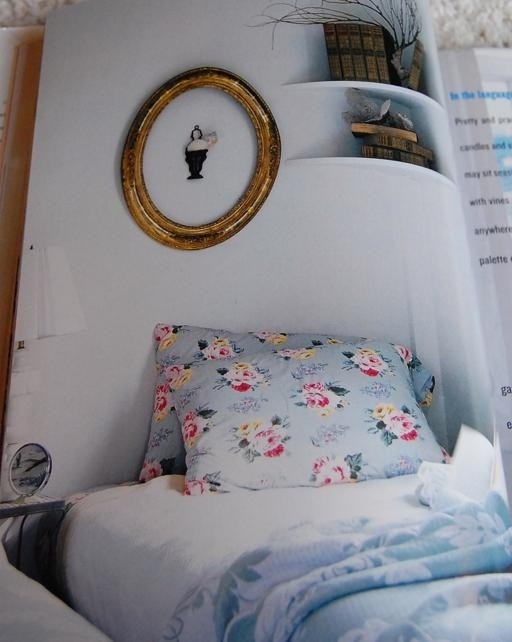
top-left (277, 73), bottom-right (458, 191)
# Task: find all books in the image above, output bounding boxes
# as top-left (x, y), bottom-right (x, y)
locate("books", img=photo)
top-left (322, 20), bottom-right (435, 169)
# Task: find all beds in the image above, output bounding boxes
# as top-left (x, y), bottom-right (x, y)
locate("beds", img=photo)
top-left (48, 451), bottom-right (511, 642)
